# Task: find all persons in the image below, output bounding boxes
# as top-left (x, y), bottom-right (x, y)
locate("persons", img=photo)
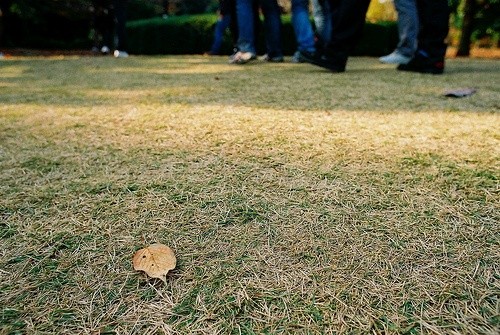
top-left (398, 0), bottom-right (450, 74)
top-left (229, 1), bottom-right (284, 63)
top-left (289, 1), bottom-right (333, 69)
top-left (220, 1), bottom-right (267, 61)
top-left (379, 0), bottom-right (418, 64)
top-left (295, 0), bottom-right (373, 73)
top-left (200, 0), bottom-right (232, 57)
top-left (94, 1), bottom-right (128, 58)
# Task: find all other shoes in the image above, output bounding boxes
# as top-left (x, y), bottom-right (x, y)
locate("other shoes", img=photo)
top-left (396, 54), bottom-right (445, 74)
top-left (377, 50), bottom-right (414, 64)
top-left (202, 43), bottom-right (348, 73)
top-left (90, 46), bottom-right (129, 58)
